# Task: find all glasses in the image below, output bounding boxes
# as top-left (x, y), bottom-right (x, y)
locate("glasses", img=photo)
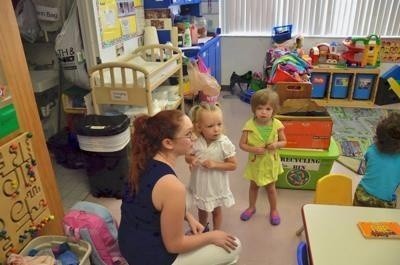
top-left (169, 134), bottom-right (194, 140)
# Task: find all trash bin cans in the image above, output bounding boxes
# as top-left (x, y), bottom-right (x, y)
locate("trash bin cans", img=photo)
top-left (73, 113), bottom-right (132, 199)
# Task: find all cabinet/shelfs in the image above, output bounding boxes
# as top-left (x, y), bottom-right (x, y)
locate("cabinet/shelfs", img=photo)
top-left (88, 44), bottom-right (186, 136)
top-left (144, 0), bottom-right (222, 103)
top-left (302, 63), bottom-right (381, 108)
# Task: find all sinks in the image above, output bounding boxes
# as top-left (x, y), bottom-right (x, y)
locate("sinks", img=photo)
top-left (28, 65), bottom-right (61, 93)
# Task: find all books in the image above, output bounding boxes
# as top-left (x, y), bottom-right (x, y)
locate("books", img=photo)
top-left (359, 221), bottom-right (400, 240)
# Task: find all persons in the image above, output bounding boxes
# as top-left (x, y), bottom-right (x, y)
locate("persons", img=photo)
top-left (354, 113), bottom-right (400, 208)
top-left (185, 101), bottom-right (239, 232)
top-left (118, 110), bottom-right (242, 264)
top-left (239, 89), bottom-right (286, 226)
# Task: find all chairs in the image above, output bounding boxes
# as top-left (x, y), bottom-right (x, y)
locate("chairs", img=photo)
top-left (297, 240), bottom-right (308, 265)
top-left (296, 173), bottom-right (353, 235)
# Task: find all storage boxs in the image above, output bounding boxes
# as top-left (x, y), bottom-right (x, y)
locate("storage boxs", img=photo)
top-left (274, 107), bottom-right (333, 150)
top-left (275, 134), bottom-right (341, 190)
top-left (12, 235), bottom-right (91, 265)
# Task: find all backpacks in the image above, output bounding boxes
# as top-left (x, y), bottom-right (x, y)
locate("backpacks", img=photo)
top-left (63, 200), bottom-right (128, 265)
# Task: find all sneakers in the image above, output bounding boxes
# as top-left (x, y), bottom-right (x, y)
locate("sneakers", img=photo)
top-left (270, 209), bottom-right (281, 225)
top-left (240, 207), bottom-right (256, 221)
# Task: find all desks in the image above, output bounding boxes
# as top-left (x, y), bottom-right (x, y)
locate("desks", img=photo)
top-left (302, 203), bottom-right (400, 265)
